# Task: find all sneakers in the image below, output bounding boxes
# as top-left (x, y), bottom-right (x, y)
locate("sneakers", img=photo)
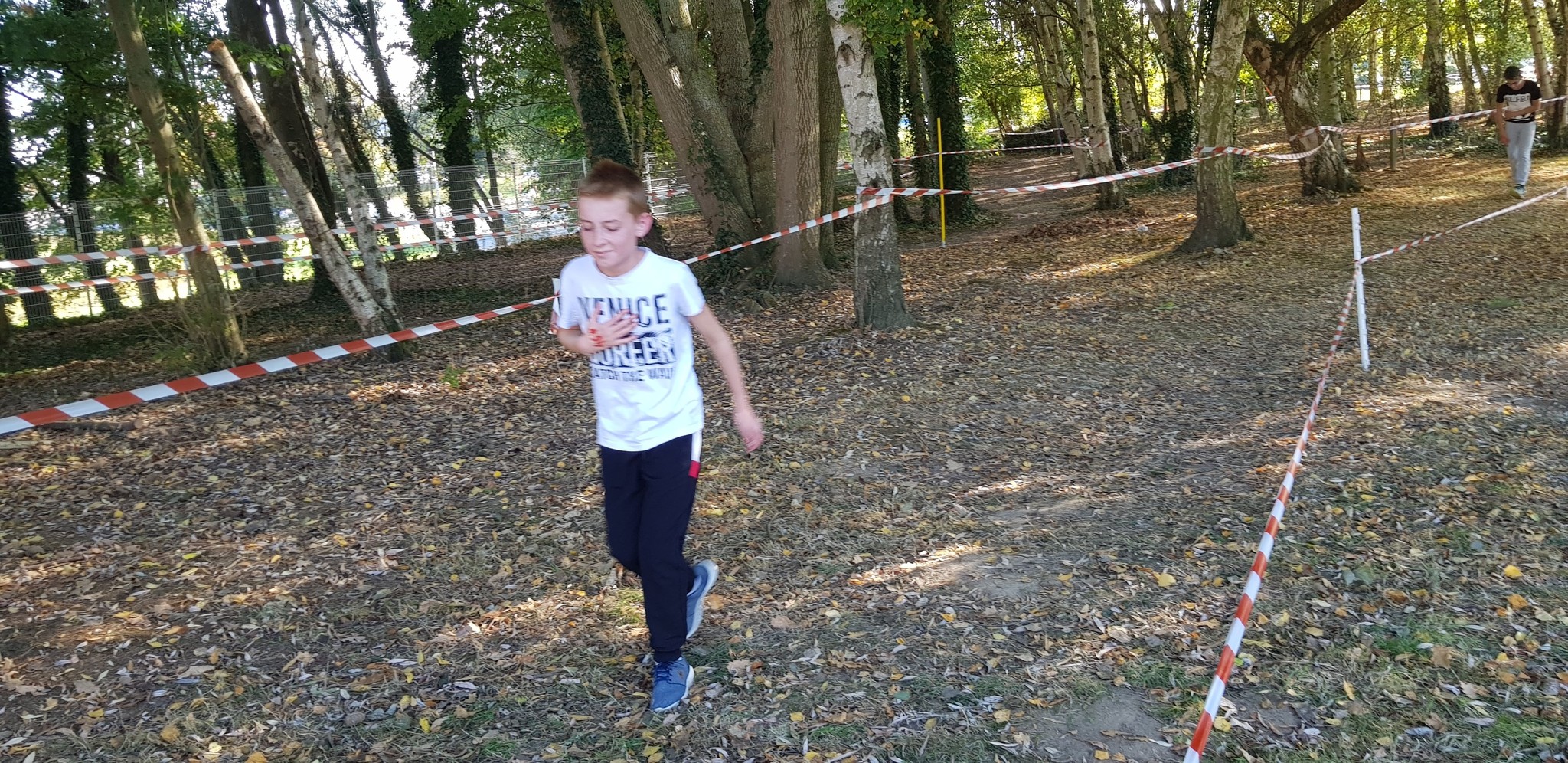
top-left (686, 560), bottom-right (719, 639)
top-left (1509, 184), bottom-right (1527, 197)
top-left (650, 652), bottom-right (695, 712)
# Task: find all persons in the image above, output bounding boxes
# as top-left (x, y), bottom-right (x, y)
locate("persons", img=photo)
top-left (1496, 67), bottom-right (1542, 196)
top-left (550, 161), bottom-right (764, 713)
